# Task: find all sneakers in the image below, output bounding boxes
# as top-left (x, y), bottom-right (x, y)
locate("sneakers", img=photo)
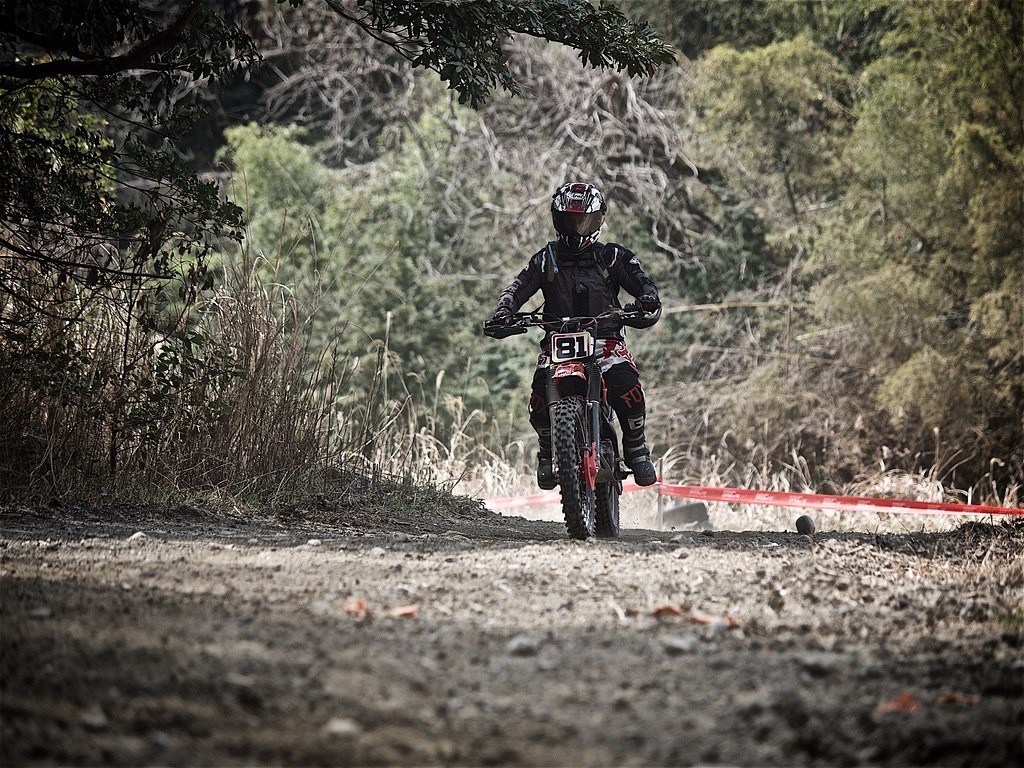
top-left (623, 443), bottom-right (656, 486)
top-left (537, 457), bottom-right (557, 490)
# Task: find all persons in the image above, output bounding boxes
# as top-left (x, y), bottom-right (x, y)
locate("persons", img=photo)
top-left (491, 181), bottom-right (660, 491)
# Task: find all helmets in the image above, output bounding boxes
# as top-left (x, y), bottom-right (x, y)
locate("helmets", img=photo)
top-left (551, 181), bottom-right (607, 255)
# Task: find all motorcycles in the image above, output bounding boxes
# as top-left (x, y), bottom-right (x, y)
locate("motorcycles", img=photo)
top-left (483, 303), bottom-right (659, 541)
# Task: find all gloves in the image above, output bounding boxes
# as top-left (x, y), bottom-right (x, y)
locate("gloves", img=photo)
top-left (492, 307), bottom-right (513, 327)
top-left (635, 295), bottom-right (659, 317)
